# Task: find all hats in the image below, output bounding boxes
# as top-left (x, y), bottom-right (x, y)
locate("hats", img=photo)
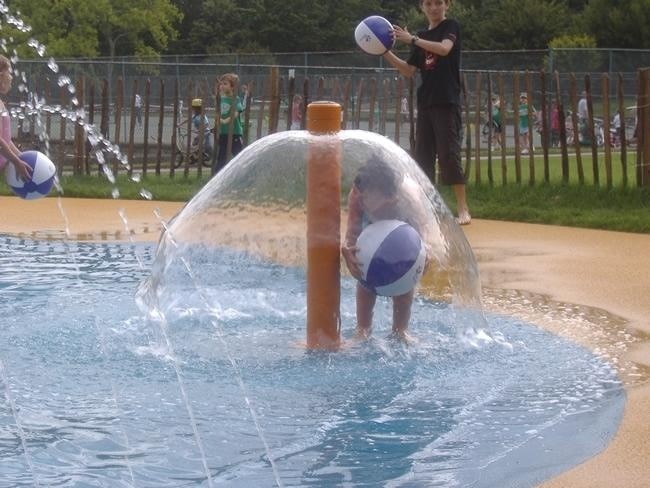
top-left (520, 92), bottom-right (528, 98)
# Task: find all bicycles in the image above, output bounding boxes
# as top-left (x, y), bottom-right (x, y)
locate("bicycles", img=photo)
top-left (566, 111), bottom-right (605, 148)
top-left (172, 125), bottom-right (213, 169)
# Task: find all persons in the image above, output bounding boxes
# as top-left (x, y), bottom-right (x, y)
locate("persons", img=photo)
top-left (379, 0), bottom-right (473, 225)
top-left (290, 94), bottom-right (303, 130)
top-left (401, 94), bottom-right (409, 112)
top-left (339, 144), bottom-right (433, 352)
top-left (0, 53), bottom-right (34, 185)
top-left (239, 84), bottom-right (254, 120)
top-left (215, 72), bottom-right (243, 172)
top-left (481, 89), bottom-right (638, 155)
top-left (134, 93), bottom-right (144, 130)
top-left (176, 98), bottom-right (212, 164)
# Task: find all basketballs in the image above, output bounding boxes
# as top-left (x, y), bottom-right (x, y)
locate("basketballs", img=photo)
top-left (355, 16), bottom-right (395, 55)
top-left (8, 150), bottom-right (55, 201)
top-left (350, 219), bottom-right (426, 297)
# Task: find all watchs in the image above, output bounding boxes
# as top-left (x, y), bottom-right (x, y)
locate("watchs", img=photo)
top-left (410, 34), bottom-right (419, 46)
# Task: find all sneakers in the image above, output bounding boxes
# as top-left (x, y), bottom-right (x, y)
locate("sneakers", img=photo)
top-left (487, 144), bottom-right (535, 154)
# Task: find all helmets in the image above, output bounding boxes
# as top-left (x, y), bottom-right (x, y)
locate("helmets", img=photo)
top-left (191, 99), bottom-right (202, 107)
